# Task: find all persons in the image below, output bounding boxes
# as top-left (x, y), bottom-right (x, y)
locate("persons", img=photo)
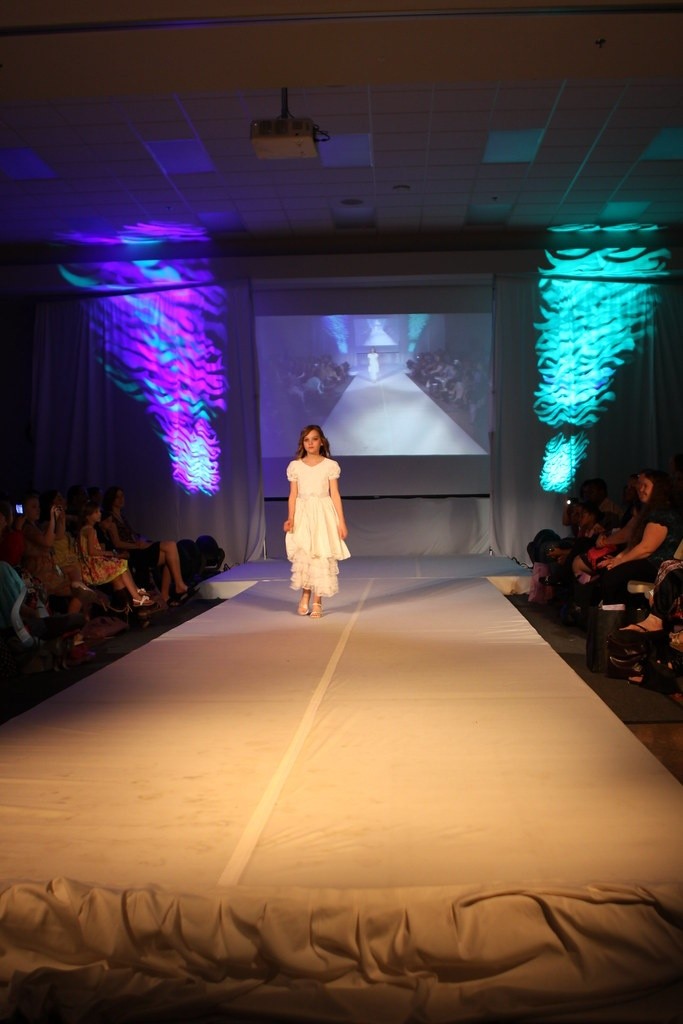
top-left (527, 456), bottom-right (683, 711)
top-left (75, 499), bottom-right (157, 609)
top-left (0, 484), bottom-right (105, 678)
top-left (367, 346), bottom-right (381, 382)
top-left (282, 425), bottom-right (352, 620)
top-left (284, 353), bottom-right (351, 405)
top-left (94, 511), bottom-right (163, 629)
top-left (405, 347), bottom-right (491, 426)
top-left (103, 486), bottom-right (200, 607)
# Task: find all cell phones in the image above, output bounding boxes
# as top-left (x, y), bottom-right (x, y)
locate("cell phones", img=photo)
top-left (12, 503), bottom-right (24, 515)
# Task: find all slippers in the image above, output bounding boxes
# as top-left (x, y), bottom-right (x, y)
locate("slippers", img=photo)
top-left (166, 598), bottom-right (180, 607)
top-left (180, 587), bottom-right (200, 604)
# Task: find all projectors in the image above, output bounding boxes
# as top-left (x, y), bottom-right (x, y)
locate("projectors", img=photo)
top-left (250, 118), bottom-right (319, 160)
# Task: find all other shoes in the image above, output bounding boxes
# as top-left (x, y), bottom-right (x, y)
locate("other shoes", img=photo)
top-left (611, 624), bottom-right (665, 645)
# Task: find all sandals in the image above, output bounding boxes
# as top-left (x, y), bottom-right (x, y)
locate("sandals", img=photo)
top-left (310, 603), bottom-right (324, 618)
top-left (297, 594), bottom-right (309, 615)
top-left (132, 596), bottom-right (156, 606)
top-left (137, 588), bottom-right (150, 596)
